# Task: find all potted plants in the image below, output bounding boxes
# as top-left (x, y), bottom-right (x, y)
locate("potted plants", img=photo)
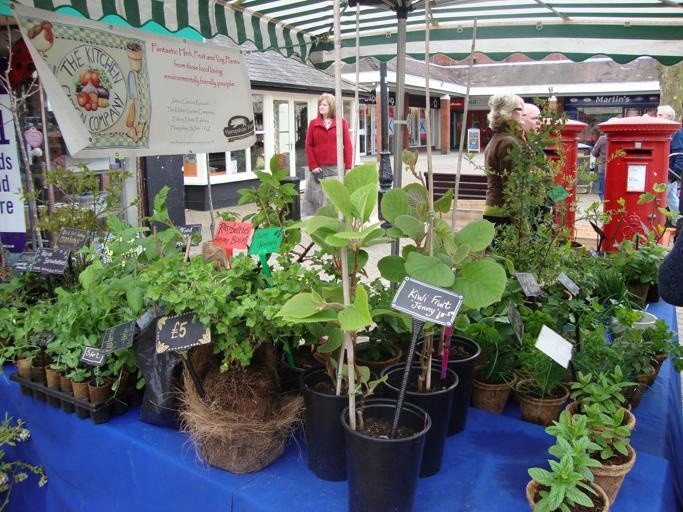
top-left (525, 365), bottom-right (637, 512)
top-left (0, 411), bottom-right (49, 512)
top-left (272, 148), bottom-right (517, 511)
top-left (463, 88), bottom-right (682, 425)
top-left (1, 152), bottom-right (336, 472)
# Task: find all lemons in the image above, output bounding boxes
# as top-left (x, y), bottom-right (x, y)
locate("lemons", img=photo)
top-left (97, 97), bottom-right (110, 107)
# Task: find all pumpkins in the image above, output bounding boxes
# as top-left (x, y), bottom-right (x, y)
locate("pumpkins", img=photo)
top-left (80, 66), bottom-right (100, 87)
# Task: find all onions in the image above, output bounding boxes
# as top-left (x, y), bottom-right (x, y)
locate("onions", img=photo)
top-left (77, 93), bottom-right (89, 106)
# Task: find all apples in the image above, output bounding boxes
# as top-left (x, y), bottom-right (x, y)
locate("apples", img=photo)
top-left (89, 93), bottom-right (98, 104)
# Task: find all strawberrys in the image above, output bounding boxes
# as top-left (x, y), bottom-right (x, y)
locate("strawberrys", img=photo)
top-left (91, 104), bottom-right (98, 110)
top-left (85, 104), bottom-right (92, 111)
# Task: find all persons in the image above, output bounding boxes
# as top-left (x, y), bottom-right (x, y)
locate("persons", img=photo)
top-left (590, 134), bottom-right (607, 202)
top-left (302, 93), bottom-right (352, 216)
top-left (521, 103), bottom-right (556, 235)
top-left (656, 105), bottom-right (683, 227)
top-left (481, 92), bottom-right (535, 248)
top-left (255, 142), bottom-right (265, 159)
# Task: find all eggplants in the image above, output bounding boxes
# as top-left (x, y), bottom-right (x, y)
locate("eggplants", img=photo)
top-left (76, 84), bottom-right (109, 98)
